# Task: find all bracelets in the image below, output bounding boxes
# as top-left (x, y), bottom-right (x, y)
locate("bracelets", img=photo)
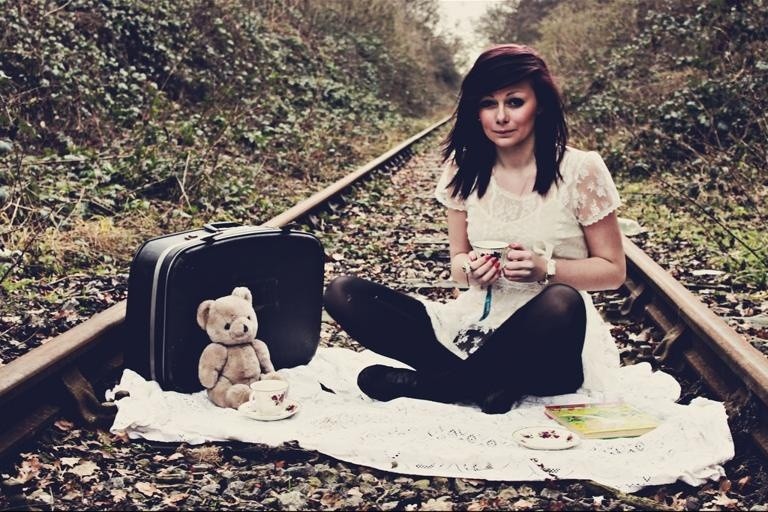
top-left (532, 239), bottom-right (557, 284)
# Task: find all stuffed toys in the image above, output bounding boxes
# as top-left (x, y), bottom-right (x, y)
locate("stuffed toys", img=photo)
top-left (199, 287), bottom-right (286, 409)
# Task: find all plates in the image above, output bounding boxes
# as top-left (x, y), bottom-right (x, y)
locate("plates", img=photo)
top-left (511, 426), bottom-right (581, 449)
top-left (237, 400), bottom-right (302, 422)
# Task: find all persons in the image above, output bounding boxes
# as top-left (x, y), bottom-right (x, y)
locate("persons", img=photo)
top-left (321, 41), bottom-right (626, 413)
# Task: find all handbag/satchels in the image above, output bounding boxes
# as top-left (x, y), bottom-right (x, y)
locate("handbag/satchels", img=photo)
top-left (123, 221), bottom-right (325, 395)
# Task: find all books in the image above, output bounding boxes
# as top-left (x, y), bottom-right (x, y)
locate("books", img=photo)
top-left (545, 401), bottom-right (661, 439)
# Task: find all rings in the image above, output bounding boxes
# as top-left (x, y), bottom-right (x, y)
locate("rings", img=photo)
top-left (462, 263), bottom-right (472, 273)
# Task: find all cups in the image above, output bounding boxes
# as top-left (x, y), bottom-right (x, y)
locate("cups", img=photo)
top-left (248, 380), bottom-right (289, 416)
top-left (472, 239), bottom-right (518, 273)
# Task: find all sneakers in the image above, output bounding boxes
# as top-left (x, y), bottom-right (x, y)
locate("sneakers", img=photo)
top-left (480, 364), bottom-right (526, 414)
top-left (357, 364), bottom-right (450, 403)
